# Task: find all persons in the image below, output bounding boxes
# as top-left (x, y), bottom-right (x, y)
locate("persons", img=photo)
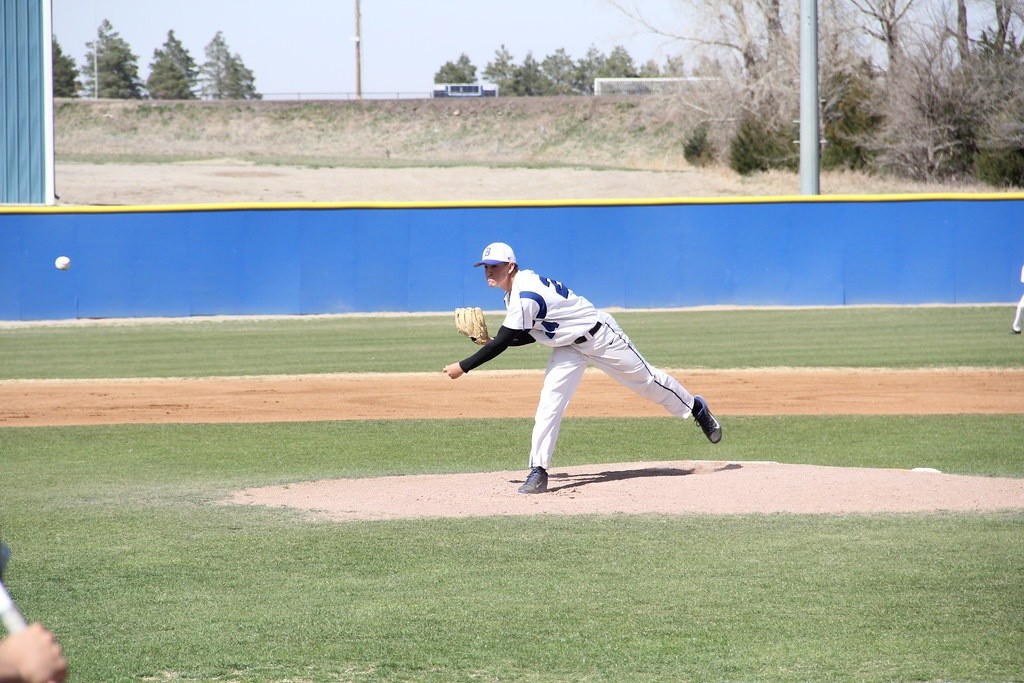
top-left (443, 242), bottom-right (721, 493)
top-left (0, 543), bottom-right (68, 682)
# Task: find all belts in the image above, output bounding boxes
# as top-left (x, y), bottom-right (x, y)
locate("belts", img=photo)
top-left (574, 322), bottom-right (603, 344)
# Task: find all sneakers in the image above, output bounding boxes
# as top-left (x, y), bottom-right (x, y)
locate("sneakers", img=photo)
top-left (692, 395), bottom-right (722, 444)
top-left (517, 468), bottom-right (548, 494)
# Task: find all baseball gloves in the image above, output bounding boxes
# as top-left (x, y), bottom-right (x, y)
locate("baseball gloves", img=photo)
top-left (454, 307), bottom-right (490, 345)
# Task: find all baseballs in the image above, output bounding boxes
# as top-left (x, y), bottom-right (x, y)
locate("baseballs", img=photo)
top-left (54, 256), bottom-right (71, 270)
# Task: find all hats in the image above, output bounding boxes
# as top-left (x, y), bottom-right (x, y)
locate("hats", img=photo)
top-left (473, 242), bottom-right (517, 266)
